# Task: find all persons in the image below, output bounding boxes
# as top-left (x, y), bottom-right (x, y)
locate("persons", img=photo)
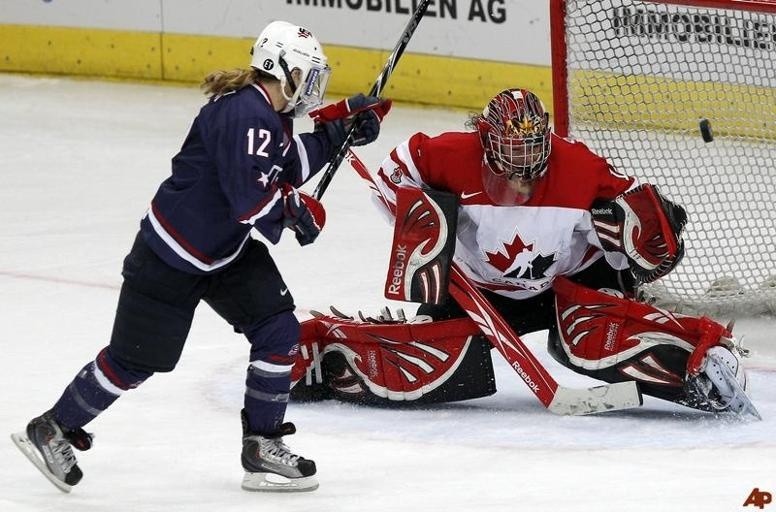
top-left (27, 17), bottom-right (392, 486)
top-left (287, 87), bottom-right (751, 417)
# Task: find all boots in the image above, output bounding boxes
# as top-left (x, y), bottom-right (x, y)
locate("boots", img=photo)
top-left (12, 408), bottom-right (93, 492)
top-left (241, 430), bottom-right (317, 493)
top-left (700, 343), bottom-right (759, 423)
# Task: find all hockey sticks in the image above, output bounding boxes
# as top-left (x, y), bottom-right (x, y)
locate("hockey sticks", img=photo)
top-left (344, 148), bottom-right (643, 415)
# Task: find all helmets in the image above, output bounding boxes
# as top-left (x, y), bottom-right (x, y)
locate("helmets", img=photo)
top-left (250, 20), bottom-right (326, 96)
top-left (477, 88), bottom-right (549, 150)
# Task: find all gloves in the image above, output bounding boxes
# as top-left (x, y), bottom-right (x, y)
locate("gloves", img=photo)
top-left (338, 96), bottom-right (392, 147)
top-left (281, 182), bottom-right (327, 247)
